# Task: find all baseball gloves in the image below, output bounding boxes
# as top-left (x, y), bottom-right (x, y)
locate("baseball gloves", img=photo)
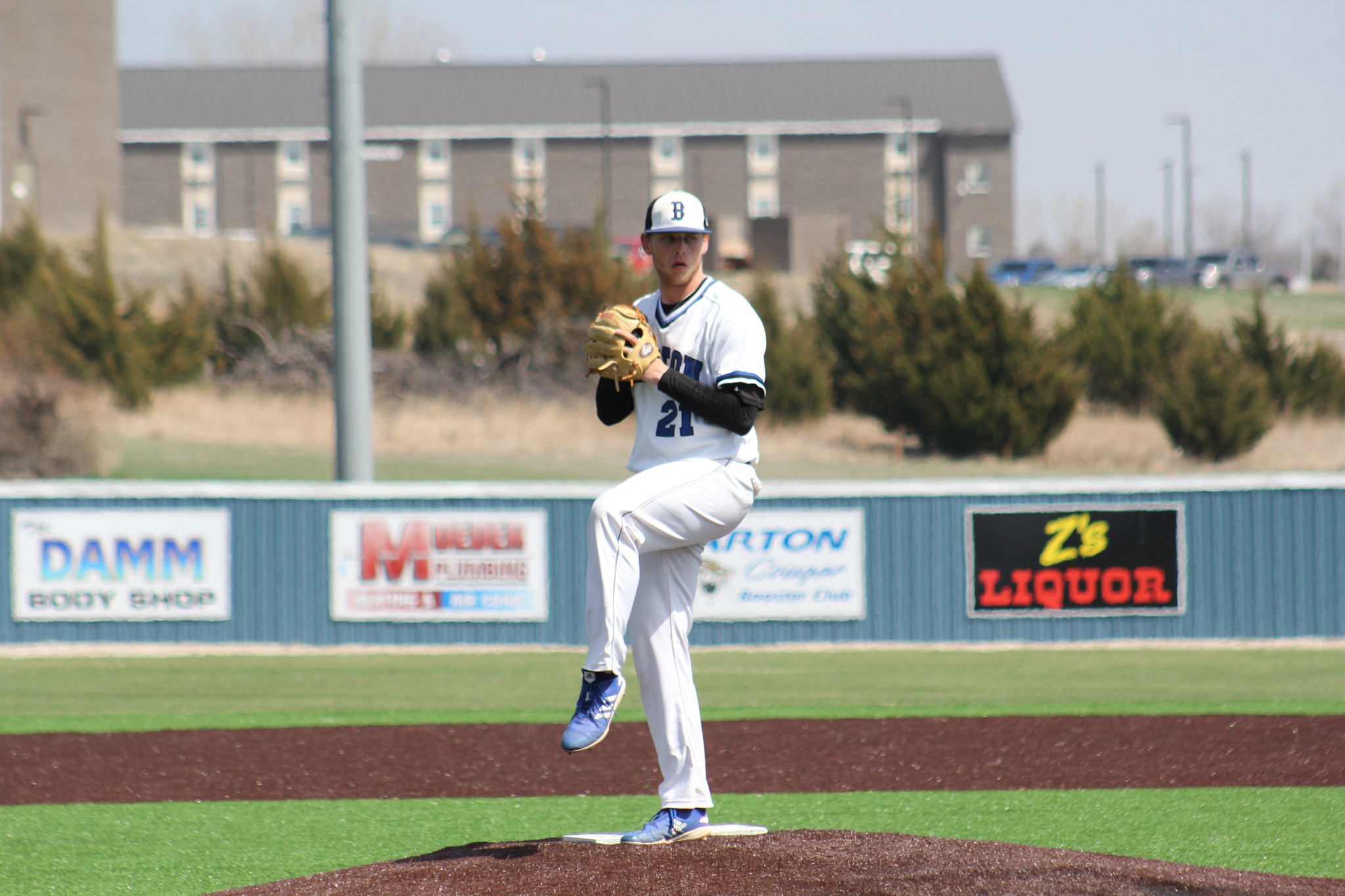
top-left (583, 302), bottom-right (663, 393)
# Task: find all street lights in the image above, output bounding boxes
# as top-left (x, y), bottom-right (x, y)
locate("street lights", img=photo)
top-left (887, 94), bottom-right (921, 248)
top-left (587, 78), bottom-right (608, 227)
top-left (1163, 109), bottom-right (1195, 263)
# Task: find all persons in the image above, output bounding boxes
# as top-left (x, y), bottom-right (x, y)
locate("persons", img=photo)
top-left (561, 190), bottom-right (766, 845)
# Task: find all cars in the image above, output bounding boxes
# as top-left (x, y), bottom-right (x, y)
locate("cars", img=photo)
top-left (992, 258), bottom-right (1197, 290)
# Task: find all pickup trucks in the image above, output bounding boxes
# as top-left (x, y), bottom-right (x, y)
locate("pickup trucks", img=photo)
top-left (1194, 251), bottom-right (1291, 289)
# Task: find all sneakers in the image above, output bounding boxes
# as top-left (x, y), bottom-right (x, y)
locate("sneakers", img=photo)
top-left (561, 668), bottom-right (626, 755)
top-left (621, 808), bottom-right (711, 844)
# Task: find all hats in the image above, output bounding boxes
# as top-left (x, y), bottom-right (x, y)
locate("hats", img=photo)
top-left (644, 189), bottom-right (712, 234)
